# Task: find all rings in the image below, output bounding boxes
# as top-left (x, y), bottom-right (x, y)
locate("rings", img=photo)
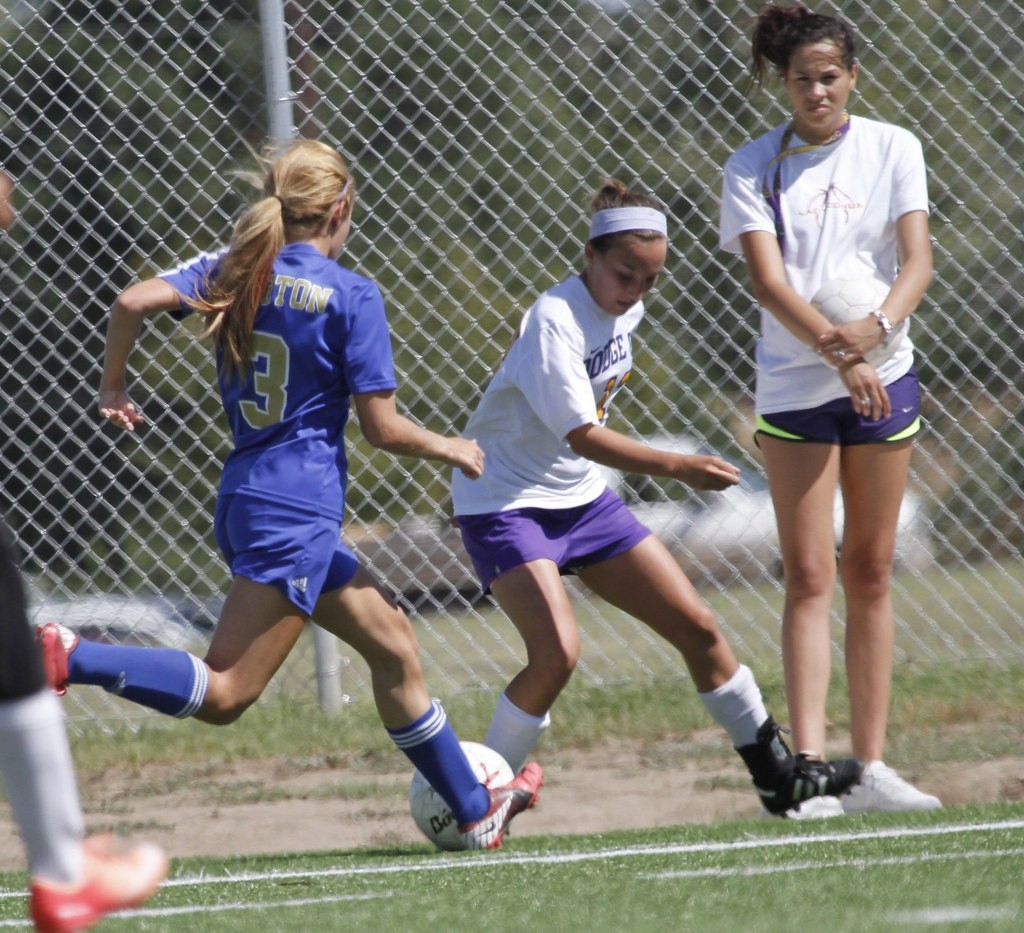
top-left (838, 351), bottom-right (845, 358)
top-left (862, 398), bottom-right (870, 408)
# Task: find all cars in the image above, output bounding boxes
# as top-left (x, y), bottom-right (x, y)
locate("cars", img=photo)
top-left (337, 430), bottom-right (940, 606)
top-left (27, 585), bottom-right (229, 662)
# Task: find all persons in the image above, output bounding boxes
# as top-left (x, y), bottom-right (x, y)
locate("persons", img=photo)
top-left (40, 141), bottom-right (546, 850)
top-left (451, 179), bottom-right (865, 818)
top-left (0, 173), bottom-right (168, 933)
top-left (721, 7), bottom-right (944, 812)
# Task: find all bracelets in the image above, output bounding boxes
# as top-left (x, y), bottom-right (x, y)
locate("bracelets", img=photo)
top-left (868, 310), bottom-right (893, 342)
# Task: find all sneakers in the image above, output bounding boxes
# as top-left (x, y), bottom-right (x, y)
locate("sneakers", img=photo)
top-left (753, 752), bottom-right (861, 819)
top-left (839, 760), bottom-right (945, 812)
top-left (457, 760), bottom-right (543, 850)
top-left (786, 793), bottom-right (847, 818)
top-left (28, 837), bottom-right (164, 933)
top-left (34, 622), bottom-right (80, 695)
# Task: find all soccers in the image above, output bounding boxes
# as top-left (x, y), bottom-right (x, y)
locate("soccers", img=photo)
top-left (408, 738), bottom-right (516, 851)
